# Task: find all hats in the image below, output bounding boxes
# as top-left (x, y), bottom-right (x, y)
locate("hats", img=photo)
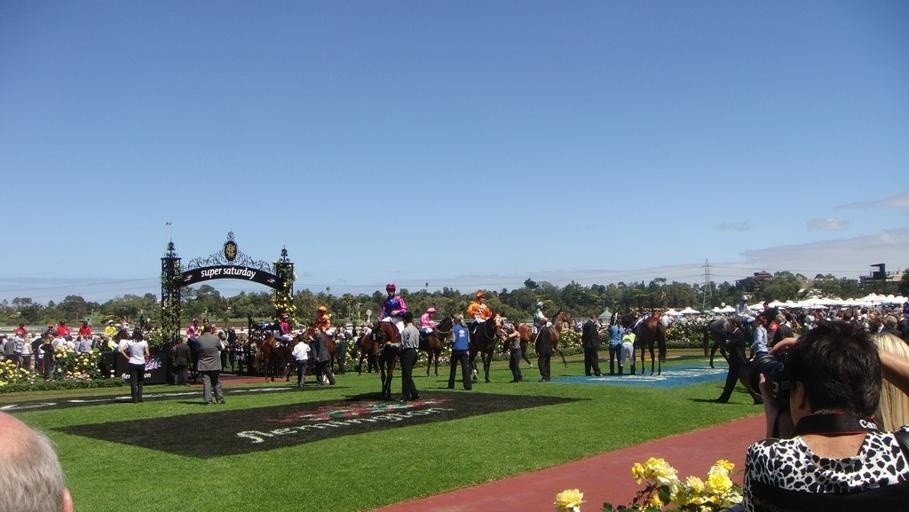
top-left (108, 320), bottom-right (114, 324)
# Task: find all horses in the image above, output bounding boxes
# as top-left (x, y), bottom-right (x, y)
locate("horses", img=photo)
top-left (260, 320), bottom-right (336, 384)
top-left (620, 315), bottom-right (666, 375)
top-left (113, 329), bottom-right (128, 343)
top-left (462, 310), bottom-right (505, 383)
top-left (418, 312), bottom-right (467, 377)
top-left (367, 321), bottom-right (404, 401)
top-left (704, 305), bottom-right (780, 369)
top-left (185, 338), bottom-right (201, 384)
top-left (351, 330), bottom-right (373, 373)
top-left (502, 306), bottom-right (570, 368)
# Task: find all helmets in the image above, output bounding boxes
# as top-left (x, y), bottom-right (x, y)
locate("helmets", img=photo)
top-left (536, 301), bottom-right (543, 307)
top-left (475, 292), bottom-right (483, 297)
top-left (319, 306), bottom-right (326, 312)
top-left (281, 314), bottom-right (288, 319)
top-left (386, 283), bottom-right (396, 290)
top-left (427, 308), bottom-right (435, 312)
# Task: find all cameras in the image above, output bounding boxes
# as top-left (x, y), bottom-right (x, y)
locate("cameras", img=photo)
top-left (752, 351), bottom-right (786, 403)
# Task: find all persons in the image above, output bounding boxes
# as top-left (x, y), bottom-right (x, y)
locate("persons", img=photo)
top-left (743, 321), bottom-right (908, 510)
top-left (1, 410), bottom-right (75, 512)
top-left (581, 311), bottom-right (637, 374)
top-left (698, 302), bottom-right (908, 405)
top-left (168, 307), bottom-right (351, 405)
top-left (0, 316), bottom-right (155, 403)
top-left (351, 282), bottom-right (555, 403)
